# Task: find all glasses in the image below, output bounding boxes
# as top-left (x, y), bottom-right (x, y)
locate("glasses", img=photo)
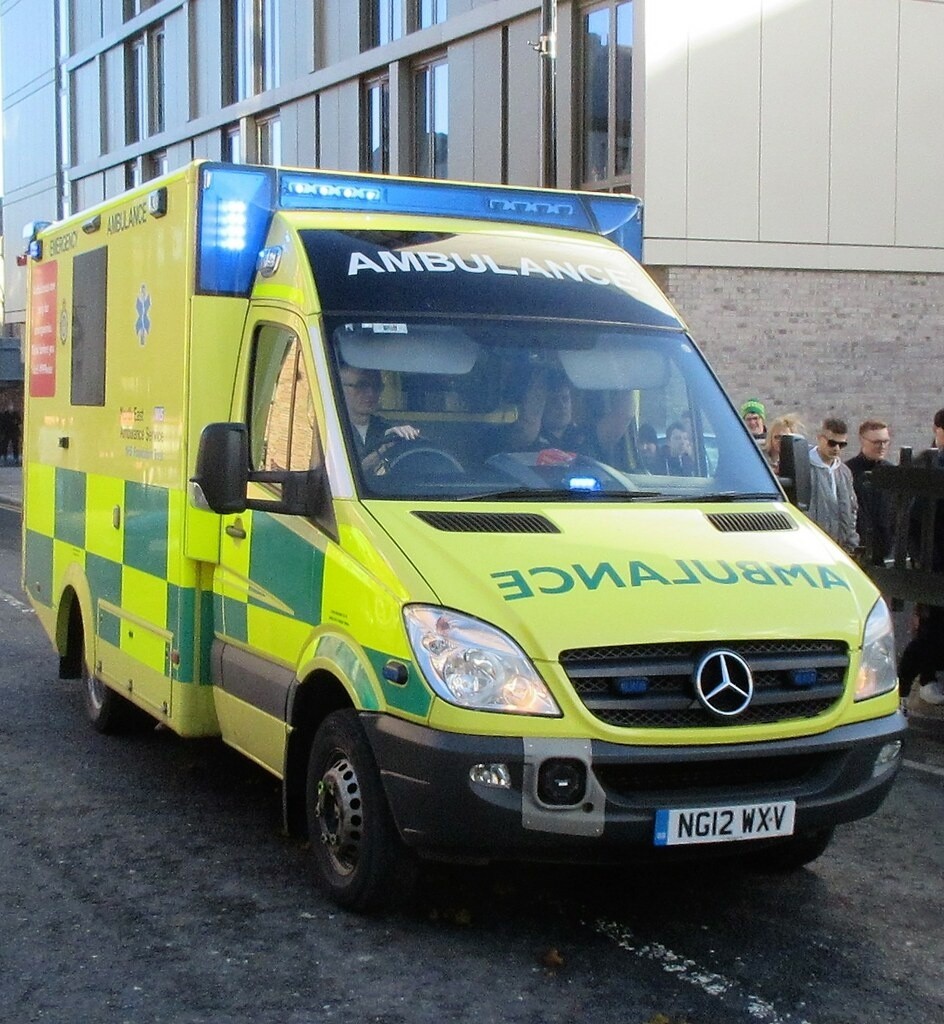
top-left (824, 436), bottom-right (847, 450)
top-left (863, 436), bottom-right (890, 445)
top-left (341, 379), bottom-right (386, 391)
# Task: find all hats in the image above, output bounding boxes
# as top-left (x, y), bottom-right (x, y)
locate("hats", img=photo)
top-left (740, 398), bottom-right (765, 420)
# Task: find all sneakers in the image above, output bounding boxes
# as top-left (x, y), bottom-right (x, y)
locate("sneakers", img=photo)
top-left (919, 682), bottom-right (944, 704)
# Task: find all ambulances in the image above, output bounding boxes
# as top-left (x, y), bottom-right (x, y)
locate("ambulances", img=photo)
top-left (21, 157), bottom-right (910, 916)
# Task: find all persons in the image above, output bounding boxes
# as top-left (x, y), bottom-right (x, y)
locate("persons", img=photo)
top-left (737, 399), bottom-right (769, 446)
top-left (792, 418), bottom-right (859, 557)
top-left (836, 419), bottom-right (898, 609)
top-left (892, 406), bottom-right (944, 712)
top-left (330, 365), bottom-right (421, 472)
top-left (759, 411), bottom-right (802, 472)
top-left (632, 403), bottom-right (705, 479)
top-left (508, 353), bottom-right (642, 455)
